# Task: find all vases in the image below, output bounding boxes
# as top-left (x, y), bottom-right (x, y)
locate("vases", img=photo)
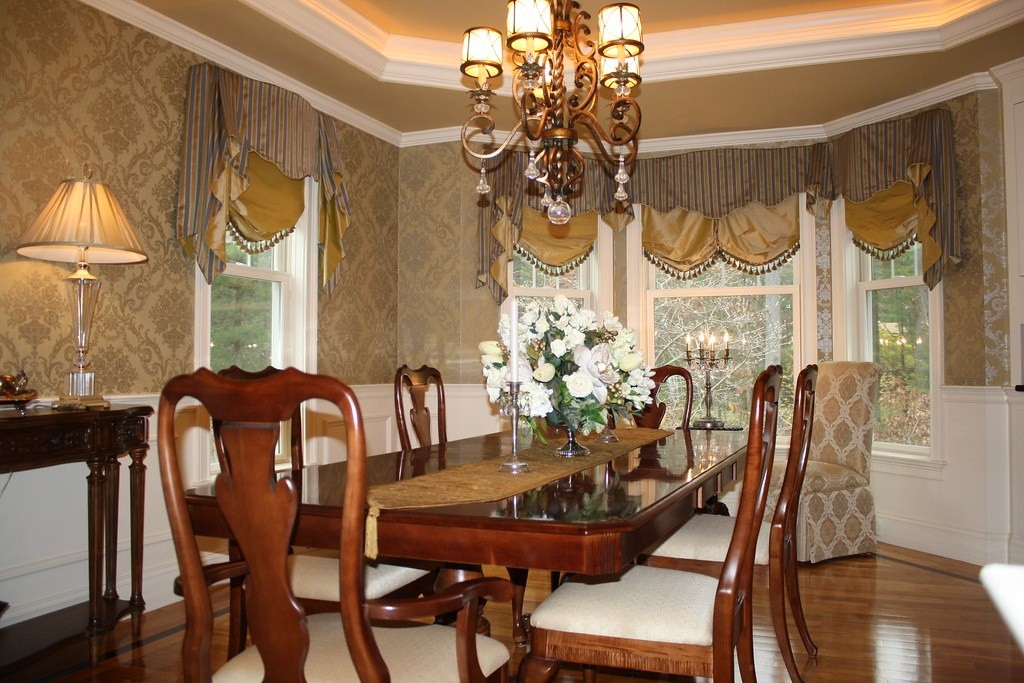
top-left (543, 405), bottom-right (591, 458)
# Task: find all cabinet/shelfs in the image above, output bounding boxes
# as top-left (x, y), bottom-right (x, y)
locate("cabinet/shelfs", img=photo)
top-left (0, 404), bottom-right (154, 679)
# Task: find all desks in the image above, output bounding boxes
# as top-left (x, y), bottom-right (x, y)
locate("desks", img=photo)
top-left (676, 425), bottom-right (743, 517)
top-left (182, 431), bottom-right (766, 637)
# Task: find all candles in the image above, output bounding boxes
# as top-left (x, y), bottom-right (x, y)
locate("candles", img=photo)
top-left (510, 295), bottom-right (519, 382)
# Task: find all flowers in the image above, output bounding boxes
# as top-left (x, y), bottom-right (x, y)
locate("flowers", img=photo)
top-left (481, 293), bottom-right (657, 446)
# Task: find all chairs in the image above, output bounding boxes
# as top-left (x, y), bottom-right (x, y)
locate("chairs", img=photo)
top-left (519, 363), bottom-right (819, 683)
top-left (157, 364), bottom-right (512, 683)
top-left (737, 361), bottom-right (884, 564)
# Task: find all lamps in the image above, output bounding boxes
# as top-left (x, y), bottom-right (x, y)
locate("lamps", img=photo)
top-left (16, 160), bottom-right (151, 409)
top-left (684, 331), bottom-right (734, 427)
top-left (458, 0), bottom-right (646, 225)
